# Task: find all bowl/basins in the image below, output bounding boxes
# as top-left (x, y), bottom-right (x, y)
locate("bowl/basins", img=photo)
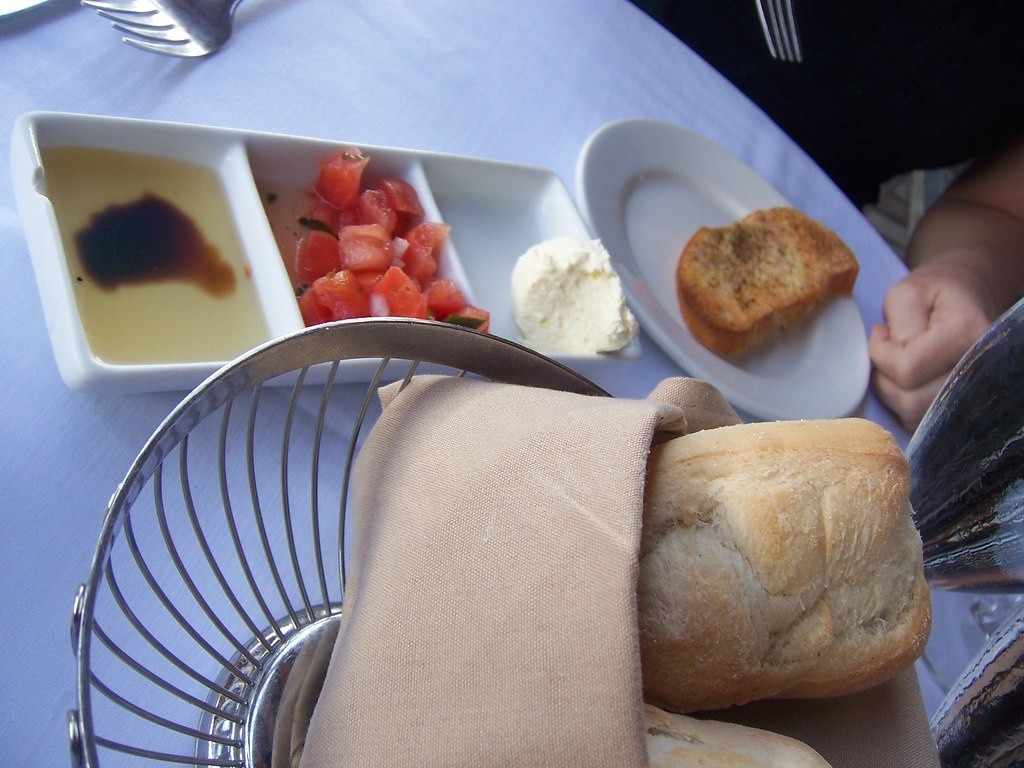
top-left (5, 110), bottom-right (644, 390)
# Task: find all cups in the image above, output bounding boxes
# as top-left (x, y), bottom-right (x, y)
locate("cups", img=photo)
top-left (901, 298), bottom-right (1024, 605)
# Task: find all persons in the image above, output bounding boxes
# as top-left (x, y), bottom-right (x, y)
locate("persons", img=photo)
top-left (636, 0), bottom-right (1024, 432)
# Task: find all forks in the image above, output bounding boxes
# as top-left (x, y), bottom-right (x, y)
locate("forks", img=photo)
top-left (80, 0), bottom-right (242, 59)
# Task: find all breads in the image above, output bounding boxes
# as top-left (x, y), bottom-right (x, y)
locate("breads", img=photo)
top-left (676, 207), bottom-right (860, 362)
top-left (643, 703), bottom-right (836, 768)
top-left (637, 417), bottom-right (933, 714)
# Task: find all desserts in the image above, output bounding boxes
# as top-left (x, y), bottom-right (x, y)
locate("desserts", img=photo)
top-left (511, 234), bottom-right (640, 355)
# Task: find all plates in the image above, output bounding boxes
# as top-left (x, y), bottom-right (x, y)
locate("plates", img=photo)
top-left (577, 116), bottom-right (875, 426)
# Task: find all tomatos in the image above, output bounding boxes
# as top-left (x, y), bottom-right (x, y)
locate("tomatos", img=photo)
top-left (293, 147), bottom-right (492, 336)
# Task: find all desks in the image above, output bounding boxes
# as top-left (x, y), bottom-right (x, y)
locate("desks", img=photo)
top-left (0, 0), bottom-right (1024, 768)
